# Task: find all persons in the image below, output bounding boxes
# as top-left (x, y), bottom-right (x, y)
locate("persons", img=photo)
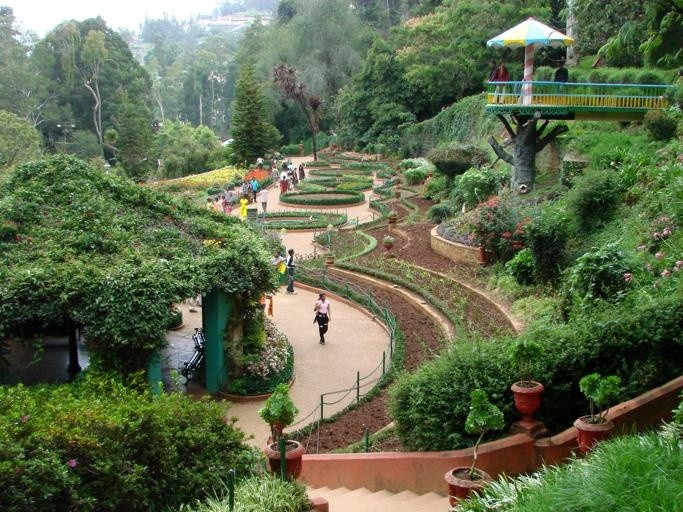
top-left (445, 103), bottom-right (449, 109)
top-left (489, 58), bottom-right (568, 105)
top-left (285, 250), bottom-right (297, 292)
top-left (314, 292), bottom-right (332, 345)
top-left (206, 151), bottom-right (305, 220)
top-left (441, 103), bottom-right (445, 110)
top-left (482, 89), bottom-right (486, 93)
top-left (270, 252), bottom-right (287, 274)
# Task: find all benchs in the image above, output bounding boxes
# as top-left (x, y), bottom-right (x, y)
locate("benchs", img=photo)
top-left (192, 326), bottom-right (205, 349)
top-left (180, 346), bottom-right (205, 386)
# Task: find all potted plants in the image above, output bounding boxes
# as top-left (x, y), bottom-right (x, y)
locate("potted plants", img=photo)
top-left (258, 383), bottom-right (305, 479)
top-left (502, 337), bottom-right (545, 428)
top-left (386, 211), bottom-right (398, 223)
top-left (324, 252), bottom-right (335, 264)
top-left (442, 388), bottom-right (505, 508)
top-left (381, 236), bottom-right (393, 251)
top-left (570, 371), bottom-right (628, 452)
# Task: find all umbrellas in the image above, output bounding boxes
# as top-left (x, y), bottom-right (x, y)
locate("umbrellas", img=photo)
top-left (486, 17), bottom-right (575, 104)
